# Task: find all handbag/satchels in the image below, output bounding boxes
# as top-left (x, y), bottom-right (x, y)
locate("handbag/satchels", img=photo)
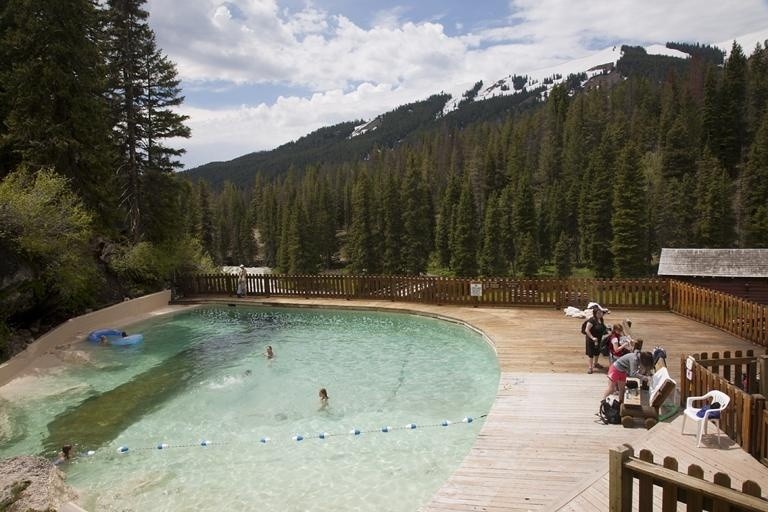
top-left (582, 320), bottom-right (594, 334)
top-left (599, 337), bottom-right (612, 356)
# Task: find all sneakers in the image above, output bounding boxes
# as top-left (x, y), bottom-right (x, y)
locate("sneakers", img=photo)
top-left (593, 363), bottom-right (602, 368)
top-left (588, 368), bottom-right (592, 373)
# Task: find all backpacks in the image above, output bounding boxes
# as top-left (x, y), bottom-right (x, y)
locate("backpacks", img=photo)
top-left (599, 399), bottom-right (620, 424)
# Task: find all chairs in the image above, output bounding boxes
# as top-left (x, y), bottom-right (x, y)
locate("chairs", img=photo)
top-left (681, 390), bottom-right (731, 448)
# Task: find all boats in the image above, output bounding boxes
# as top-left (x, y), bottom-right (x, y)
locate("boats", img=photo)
top-left (89, 329), bottom-right (144, 347)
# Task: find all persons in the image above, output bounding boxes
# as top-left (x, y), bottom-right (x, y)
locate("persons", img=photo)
top-left (319, 388), bottom-right (328, 401)
top-left (607, 324), bottom-right (630, 357)
top-left (59, 445), bottom-right (72, 461)
top-left (603, 351), bottom-right (655, 403)
top-left (264, 345), bottom-right (276, 358)
top-left (237, 264), bottom-right (247, 297)
top-left (122, 332), bottom-right (126, 337)
top-left (585, 308), bottom-right (612, 373)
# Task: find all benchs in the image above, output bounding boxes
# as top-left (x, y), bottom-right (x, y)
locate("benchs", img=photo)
top-left (619, 348), bottom-right (674, 429)
